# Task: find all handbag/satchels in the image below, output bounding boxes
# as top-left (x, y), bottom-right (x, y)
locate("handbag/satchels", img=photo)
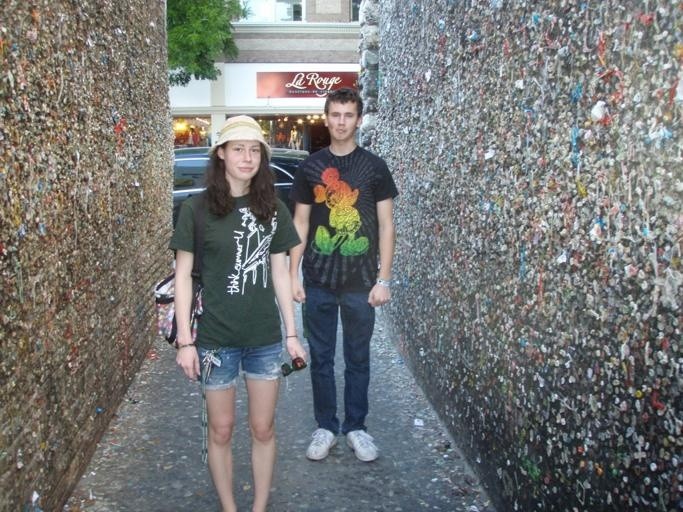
top-left (153, 193), bottom-right (206, 350)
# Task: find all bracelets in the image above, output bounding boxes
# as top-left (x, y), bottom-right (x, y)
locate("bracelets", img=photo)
top-left (378, 280), bottom-right (391, 286)
top-left (177, 343), bottom-right (195, 348)
top-left (287, 336), bottom-right (297, 337)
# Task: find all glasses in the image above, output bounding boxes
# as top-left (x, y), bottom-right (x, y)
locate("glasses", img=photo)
top-left (280, 357), bottom-right (308, 378)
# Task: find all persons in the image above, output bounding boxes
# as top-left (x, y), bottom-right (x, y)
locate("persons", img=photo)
top-left (168, 115), bottom-right (308, 512)
top-left (290, 88), bottom-right (399, 461)
top-left (291, 123), bottom-right (301, 150)
top-left (187, 128), bottom-right (198, 147)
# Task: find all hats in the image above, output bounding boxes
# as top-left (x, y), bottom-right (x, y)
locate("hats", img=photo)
top-left (207, 114), bottom-right (272, 162)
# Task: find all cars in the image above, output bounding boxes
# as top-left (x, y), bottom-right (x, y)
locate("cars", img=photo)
top-left (173, 146), bottom-right (312, 260)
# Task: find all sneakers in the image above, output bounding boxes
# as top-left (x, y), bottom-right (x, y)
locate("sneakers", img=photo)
top-left (306, 428), bottom-right (338, 461)
top-left (345, 428), bottom-right (380, 462)
